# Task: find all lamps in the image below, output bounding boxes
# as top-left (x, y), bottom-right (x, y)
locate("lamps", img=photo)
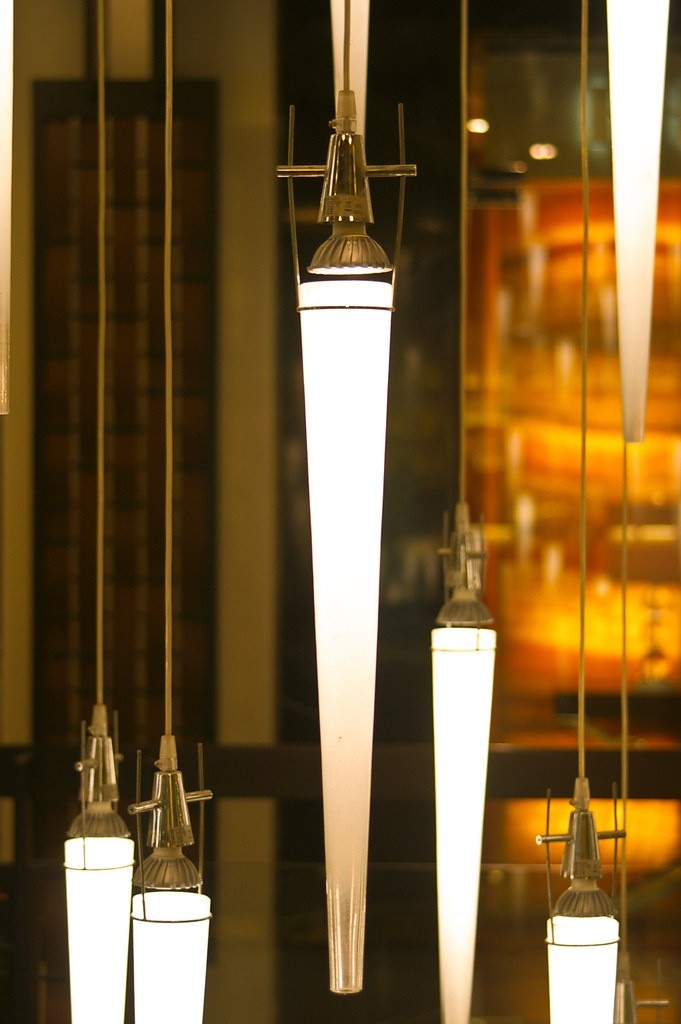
top-left (65, 1), bottom-right (635, 1023)
